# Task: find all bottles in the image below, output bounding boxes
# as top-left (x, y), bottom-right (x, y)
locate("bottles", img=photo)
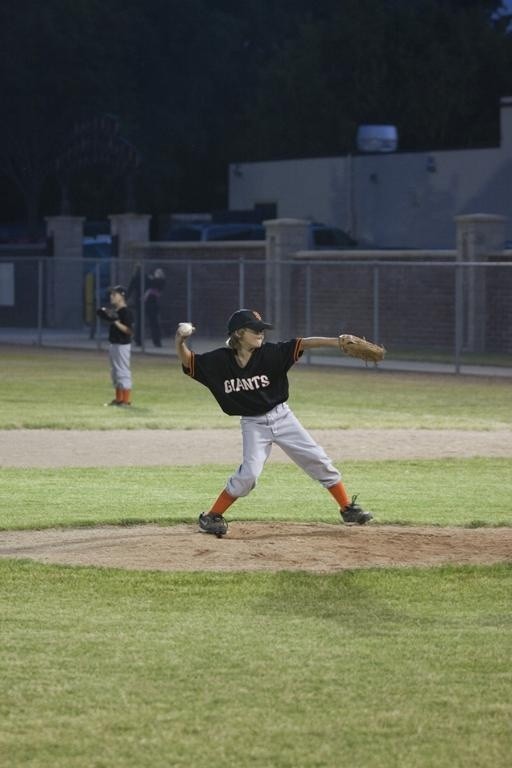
top-left (108, 285), bottom-right (127, 298)
top-left (226, 309), bottom-right (274, 331)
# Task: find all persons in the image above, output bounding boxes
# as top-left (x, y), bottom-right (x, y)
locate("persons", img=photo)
top-left (174, 309), bottom-right (386, 535)
top-left (94, 285), bottom-right (135, 408)
top-left (126, 261), bottom-right (170, 349)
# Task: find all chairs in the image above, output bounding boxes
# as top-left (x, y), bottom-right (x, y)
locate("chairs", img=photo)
top-left (103, 399), bottom-right (131, 407)
top-left (199, 511), bottom-right (228, 535)
top-left (339, 494), bottom-right (374, 526)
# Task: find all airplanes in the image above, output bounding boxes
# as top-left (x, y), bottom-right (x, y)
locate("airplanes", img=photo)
top-left (98, 307), bottom-right (120, 321)
top-left (339, 334), bottom-right (384, 360)
